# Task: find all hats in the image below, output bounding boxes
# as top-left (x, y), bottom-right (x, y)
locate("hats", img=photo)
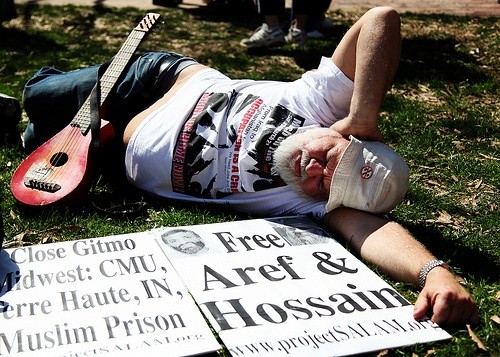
top-left (324, 135), bottom-right (411, 217)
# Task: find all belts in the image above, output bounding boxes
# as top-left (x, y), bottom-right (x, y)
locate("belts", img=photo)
top-left (141, 55), bottom-right (180, 93)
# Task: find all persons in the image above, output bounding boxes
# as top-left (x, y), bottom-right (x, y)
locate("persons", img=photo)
top-left (161, 229), bottom-right (209, 256)
top-left (21, 5), bottom-right (476, 327)
top-left (239, 0), bottom-right (333, 48)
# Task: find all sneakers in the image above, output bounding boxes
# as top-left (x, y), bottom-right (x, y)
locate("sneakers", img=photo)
top-left (282, 25), bottom-right (330, 44)
top-left (240, 24), bottom-right (287, 50)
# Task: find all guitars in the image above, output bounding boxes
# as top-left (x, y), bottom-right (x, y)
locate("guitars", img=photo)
top-left (10, 12), bottom-right (167, 208)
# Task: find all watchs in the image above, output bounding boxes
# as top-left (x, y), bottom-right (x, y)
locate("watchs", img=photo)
top-left (418, 260), bottom-right (453, 287)
